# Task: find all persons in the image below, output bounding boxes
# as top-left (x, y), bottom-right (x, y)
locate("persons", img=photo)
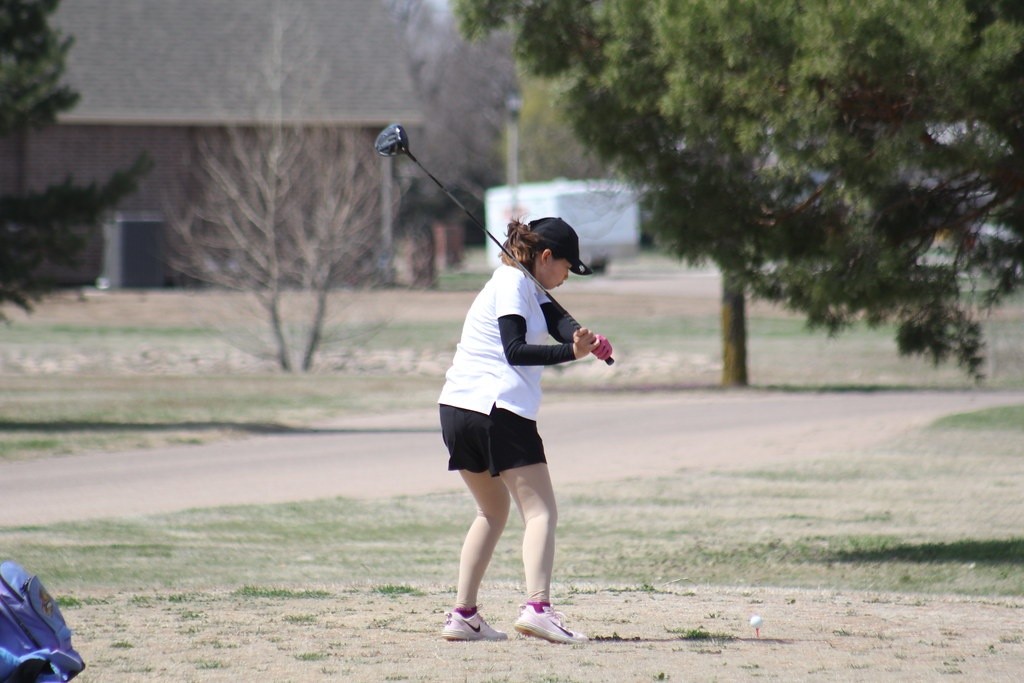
top-left (437, 216), bottom-right (612, 644)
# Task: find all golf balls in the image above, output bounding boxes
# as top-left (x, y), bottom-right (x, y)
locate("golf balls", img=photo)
top-left (751, 616), bottom-right (763, 629)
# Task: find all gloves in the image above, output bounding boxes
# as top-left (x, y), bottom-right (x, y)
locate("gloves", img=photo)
top-left (591, 332), bottom-right (613, 361)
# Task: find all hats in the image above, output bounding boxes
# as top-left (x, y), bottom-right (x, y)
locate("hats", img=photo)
top-left (525, 217), bottom-right (593, 276)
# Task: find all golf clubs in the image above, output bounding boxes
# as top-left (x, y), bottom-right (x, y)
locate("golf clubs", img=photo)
top-left (375, 124), bottom-right (615, 367)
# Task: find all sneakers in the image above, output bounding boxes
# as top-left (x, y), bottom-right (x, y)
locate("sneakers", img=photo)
top-left (516, 604), bottom-right (592, 645)
top-left (440, 611), bottom-right (508, 641)
